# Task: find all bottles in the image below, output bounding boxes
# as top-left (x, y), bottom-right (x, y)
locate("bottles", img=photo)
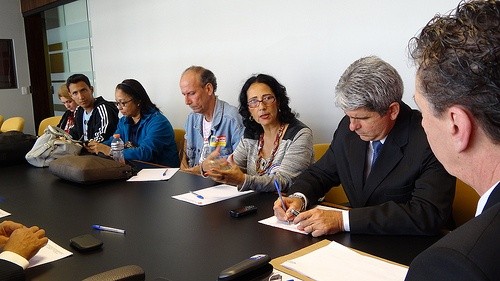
top-left (111, 133), bottom-right (125, 163)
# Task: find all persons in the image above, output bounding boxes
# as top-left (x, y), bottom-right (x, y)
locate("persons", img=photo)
top-left (403, 0), bottom-right (500, 281)
top-left (0, 221), bottom-right (48, 281)
top-left (179, 66), bottom-right (245, 174)
top-left (273, 55), bottom-right (456, 238)
top-left (65, 74), bottom-right (120, 145)
top-left (84, 78), bottom-right (179, 168)
top-left (58, 84), bottom-right (78, 136)
top-left (204, 73), bottom-right (315, 194)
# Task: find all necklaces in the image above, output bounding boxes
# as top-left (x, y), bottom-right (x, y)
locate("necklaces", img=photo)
top-left (256, 124), bottom-right (285, 176)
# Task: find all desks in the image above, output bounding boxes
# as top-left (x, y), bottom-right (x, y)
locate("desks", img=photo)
top-left (0, 135), bottom-right (450, 281)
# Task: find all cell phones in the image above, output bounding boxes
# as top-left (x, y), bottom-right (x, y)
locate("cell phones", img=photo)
top-left (291, 208), bottom-right (308, 221)
top-left (231, 205), bottom-right (258, 217)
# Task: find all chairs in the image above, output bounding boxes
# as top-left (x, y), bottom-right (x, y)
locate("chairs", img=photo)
top-left (38, 115), bottom-right (62, 138)
top-left (1, 117), bottom-right (25, 133)
top-left (452, 178), bottom-right (480, 224)
top-left (312, 143), bottom-right (349, 204)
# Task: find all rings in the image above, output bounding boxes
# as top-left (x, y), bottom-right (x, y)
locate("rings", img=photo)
top-left (222, 176), bottom-right (224, 180)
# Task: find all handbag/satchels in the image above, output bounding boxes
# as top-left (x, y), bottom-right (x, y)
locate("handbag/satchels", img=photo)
top-left (24, 124), bottom-right (83, 168)
top-left (47, 155), bottom-right (143, 185)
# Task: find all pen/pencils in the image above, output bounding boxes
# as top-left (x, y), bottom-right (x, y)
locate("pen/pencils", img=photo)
top-left (163, 168), bottom-right (169, 176)
top-left (189, 190), bottom-right (204, 199)
top-left (274, 179), bottom-right (290, 224)
top-left (91, 224), bottom-right (126, 234)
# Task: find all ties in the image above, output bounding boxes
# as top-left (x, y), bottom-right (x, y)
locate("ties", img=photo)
top-left (371, 140), bottom-right (383, 168)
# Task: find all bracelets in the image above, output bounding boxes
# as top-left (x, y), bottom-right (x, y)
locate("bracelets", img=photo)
top-left (292, 194), bottom-right (305, 211)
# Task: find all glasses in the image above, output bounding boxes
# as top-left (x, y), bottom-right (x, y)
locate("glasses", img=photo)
top-left (114, 98), bottom-right (135, 106)
top-left (246, 95), bottom-right (276, 109)
